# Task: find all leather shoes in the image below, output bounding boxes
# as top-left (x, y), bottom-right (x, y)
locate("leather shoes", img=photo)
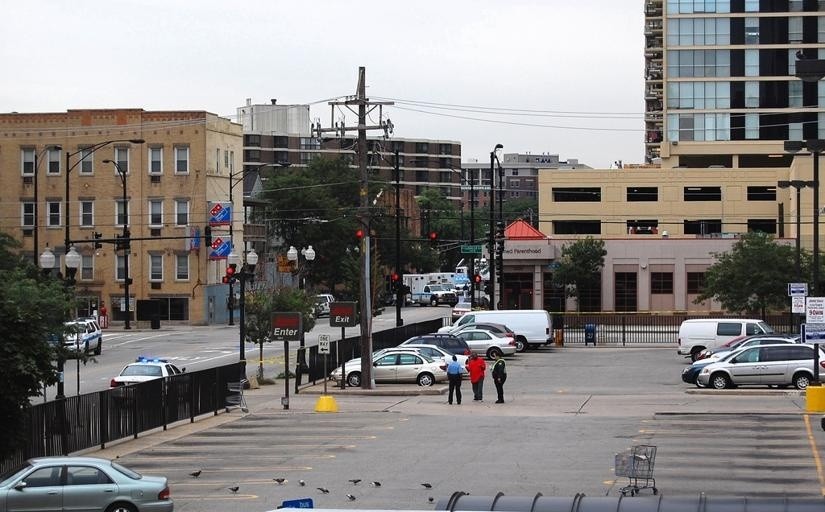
top-left (495, 400), bottom-right (504, 404)
top-left (473, 398), bottom-right (481, 400)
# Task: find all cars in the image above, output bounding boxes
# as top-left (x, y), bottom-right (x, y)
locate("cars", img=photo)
top-left (111, 356), bottom-right (186, 405)
top-left (0, 455), bottom-right (175, 512)
top-left (327, 324), bottom-right (520, 386)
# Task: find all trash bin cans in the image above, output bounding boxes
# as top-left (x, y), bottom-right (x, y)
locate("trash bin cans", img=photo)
top-left (150, 313), bottom-right (160, 329)
top-left (585, 324), bottom-right (596, 346)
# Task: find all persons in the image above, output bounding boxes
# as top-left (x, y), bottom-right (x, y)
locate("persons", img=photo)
top-left (465, 352), bottom-right (486, 401)
top-left (447, 356), bottom-right (462, 405)
top-left (491, 352), bottom-right (506, 404)
top-left (99, 300), bottom-right (107, 316)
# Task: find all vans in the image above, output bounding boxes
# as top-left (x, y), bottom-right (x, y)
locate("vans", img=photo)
top-left (677, 318), bottom-right (773, 362)
top-left (682, 331), bottom-right (824, 391)
top-left (310, 294), bottom-right (335, 317)
top-left (49, 317), bottom-right (102, 358)
top-left (454, 309), bottom-right (552, 352)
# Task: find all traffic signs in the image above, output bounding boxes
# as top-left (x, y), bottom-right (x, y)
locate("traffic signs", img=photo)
top-left (462, 245), bottom-right (482, 254)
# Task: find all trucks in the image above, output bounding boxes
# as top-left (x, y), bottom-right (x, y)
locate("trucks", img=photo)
top-left (401, 272), bottom-right (472, 307)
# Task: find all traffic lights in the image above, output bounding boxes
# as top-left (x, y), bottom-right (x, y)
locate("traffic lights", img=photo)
top-left (356, 229), bottom-right (363, 238)
top-left (392, 272), bottom-right (400, 289)
top-left (431, 232), bottom-right (438, 238)
top-left (205, 225), bottom-right (213, 248)
top-left (223, 267), bottom-right (236, 285)
top-left (474, 275), bottom-right (481, 290)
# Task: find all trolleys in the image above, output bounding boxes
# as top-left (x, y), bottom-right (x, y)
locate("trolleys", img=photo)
top-left (616, 444), bottom-right (659, 496)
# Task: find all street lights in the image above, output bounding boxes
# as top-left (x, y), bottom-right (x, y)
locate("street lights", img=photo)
top-left (33, 145), bottom-right (62, 264)
top-left (487, 145), bottom-right (505, 310)
top-left (287, 245), bottom-right (315, 375)
top-left (783, 140), bottom-right (825, 385)
top-left (411, 160), bottom-right (475, 311)
top-left (228, 248), bottom-right (258, 389)
top-left (228, 159), bottom-right (292, 326)
top-left (460, 200), bottom-right (479, 256)
top-left (102, 158), bottom-right (133, 331)
top-left (38, 247), bottom-right (80, 453)
top-left (318, 135), bottom-right (405, 327)
top-left (64, 139), bottom-right (144, 321)
top-left (777, 180), bottom-right (818, 332)
